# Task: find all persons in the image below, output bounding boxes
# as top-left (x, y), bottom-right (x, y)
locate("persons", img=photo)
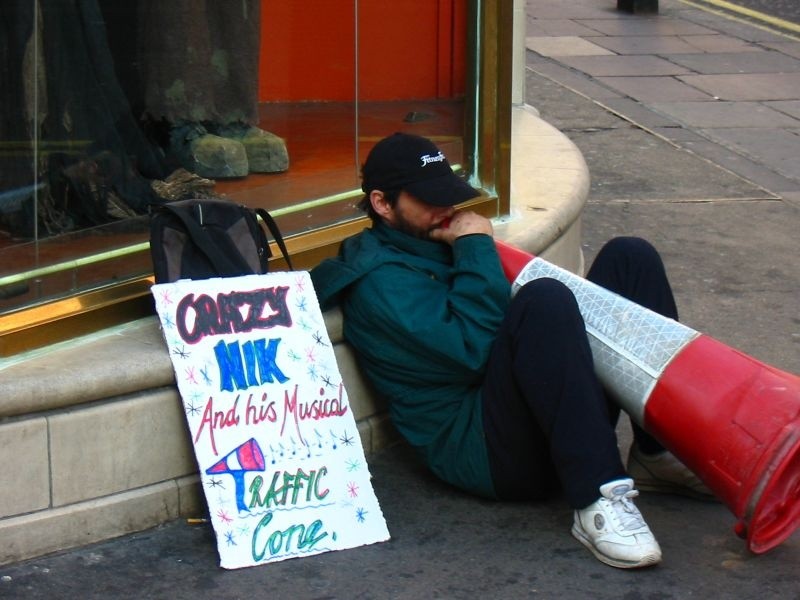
top-left (312, 133), bottom-right (722, 569)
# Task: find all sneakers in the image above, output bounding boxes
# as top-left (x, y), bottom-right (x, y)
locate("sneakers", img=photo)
top-left (624, 437), bottom-right (726, 504)
top-left (571, 478), bottom-right (663, 569)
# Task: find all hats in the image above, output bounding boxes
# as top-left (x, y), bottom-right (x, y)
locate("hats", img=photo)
top-left (362, 130), bottom-right (482, 207)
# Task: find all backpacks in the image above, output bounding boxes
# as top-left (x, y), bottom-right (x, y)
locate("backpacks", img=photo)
top-left (150, 198), bottom-right (294, 285)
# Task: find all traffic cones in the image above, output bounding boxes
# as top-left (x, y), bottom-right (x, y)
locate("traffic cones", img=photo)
top-left (438, 214), bottom-right (800, 557)
